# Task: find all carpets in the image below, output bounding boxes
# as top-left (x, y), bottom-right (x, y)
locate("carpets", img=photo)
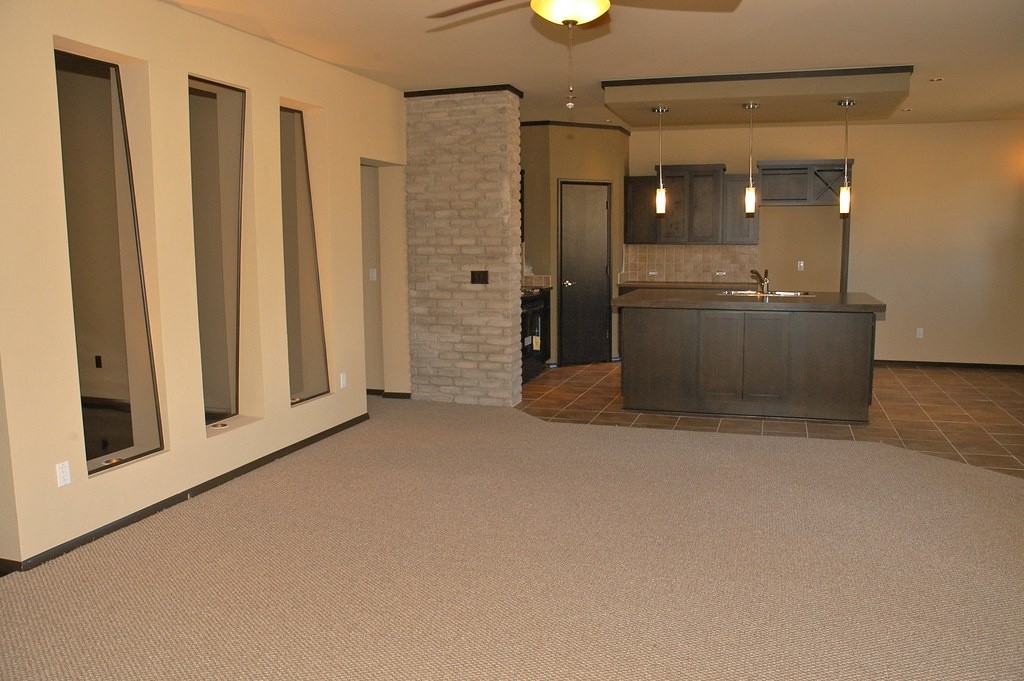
top-left (0, 395), bottom-right (1024, 681)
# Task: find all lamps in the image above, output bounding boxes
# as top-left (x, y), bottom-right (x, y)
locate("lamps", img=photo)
top-left (651, 106), bottom-right (671, 213)
top-left (838, 98), bottom-right (856, 219)
top-left (741, 101), bottom-right (760, 217)
top-left (530, 0), bottom-right (611, 108)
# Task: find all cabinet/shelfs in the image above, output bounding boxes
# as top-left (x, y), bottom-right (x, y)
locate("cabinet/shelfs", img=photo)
top-left (622, 157), bottom-right (852, 244)
top-left (616, 281), bottom-right (763, 359)
top-left (610, 312), bottom-right (887, 425)
top-left (521, 285), bottom-right (554, 383)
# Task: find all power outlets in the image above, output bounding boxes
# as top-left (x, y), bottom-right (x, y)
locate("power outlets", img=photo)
top-left (649, 271), bottom-right (659, 276)
top-left (56, 461), bottom-right (71, 488)
top-left (341, 372), bottom-right (347, 389)
top-left (716, 271), bottom-right (726, 276)
top-left (916, 328), bottom-right (924, 339)
top-left (369, 268), bottom-right (376, 281)
top-left (798, 260), bottom-right (804, 271)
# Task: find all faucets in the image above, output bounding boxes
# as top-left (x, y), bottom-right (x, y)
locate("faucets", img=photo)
top-left (750, 267), bottom-right (770, 293)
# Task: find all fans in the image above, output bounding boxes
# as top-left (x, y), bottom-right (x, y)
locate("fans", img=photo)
top-left (425, 0), bottom-right (742, 23)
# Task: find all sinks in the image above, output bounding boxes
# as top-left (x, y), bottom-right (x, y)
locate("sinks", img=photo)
top-left (772, 290), bottom-right (816, 297)
top-left (716, 289), bottom-right (758, 297)
